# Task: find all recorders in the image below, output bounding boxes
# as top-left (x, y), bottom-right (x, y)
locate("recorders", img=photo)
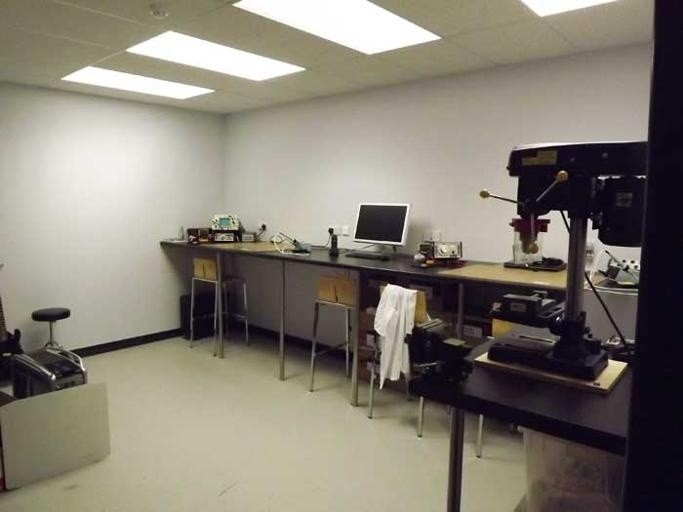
top-left (188, 228), bottom-right (212, 243)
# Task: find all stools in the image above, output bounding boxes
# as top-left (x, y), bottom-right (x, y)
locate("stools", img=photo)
top-left (31, 307), bottom-right (84, 370)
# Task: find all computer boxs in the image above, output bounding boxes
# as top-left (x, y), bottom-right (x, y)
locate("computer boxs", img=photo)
top-left (180, 291), bottom-right (232, 341)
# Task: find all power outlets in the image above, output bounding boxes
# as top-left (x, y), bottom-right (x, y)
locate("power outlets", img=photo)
top-left (257, 224), bottom-right (268, 233)
top-left (328, 225), bottom-right (339, 235)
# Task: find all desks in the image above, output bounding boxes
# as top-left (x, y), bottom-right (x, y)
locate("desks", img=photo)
top-left (408, 338), bottom-right (633, 512)
top-left (161, 238), bottom-right (567, 413)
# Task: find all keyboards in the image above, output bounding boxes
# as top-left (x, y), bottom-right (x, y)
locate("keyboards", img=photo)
top-left (346, 252), bottom-right (394, 261)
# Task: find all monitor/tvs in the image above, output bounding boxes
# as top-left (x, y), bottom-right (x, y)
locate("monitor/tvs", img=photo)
top-left (353, 203), bottom-right (412, 255)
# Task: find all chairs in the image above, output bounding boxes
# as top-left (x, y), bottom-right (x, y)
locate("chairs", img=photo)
top-left (475, 316), bottom-right (519, 458)
top-left (367, 284), bottom-right (452, 436)
top-left (308, 273), bottom-right (358, 392)
top-left (190, 257), bottom-right (250, 357)
